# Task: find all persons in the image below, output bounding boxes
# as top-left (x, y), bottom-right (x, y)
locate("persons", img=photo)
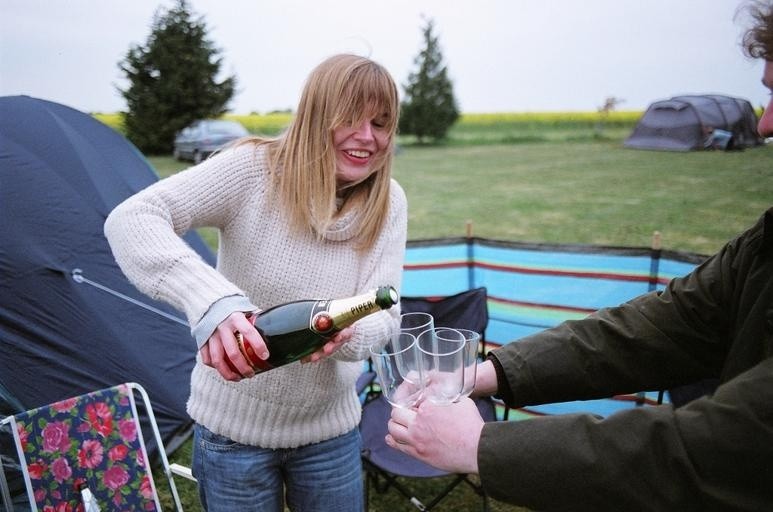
top-left (100, 51), bottom-right (408, 511)
top-left (703, 126), bottom-right (746, 153)
top-left (385, 0), bottom-right (773, 510)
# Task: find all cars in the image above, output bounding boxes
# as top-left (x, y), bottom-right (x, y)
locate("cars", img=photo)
top-left (172, 119), bottom-right (249, 165)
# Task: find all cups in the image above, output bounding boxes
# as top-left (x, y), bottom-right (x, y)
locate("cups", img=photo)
top-left (415, 326), bottom-right (467, 407)
top-left (368, 334), bottom-right (422, 409)
top-left (433, 330), bottom-right (479, 399)
top-left (388, 310), bottom-right (438, 388)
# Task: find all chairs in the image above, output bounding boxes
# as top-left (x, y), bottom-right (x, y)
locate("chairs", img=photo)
top-left (355, 286), bottom-right (497, 511)
top-left (1, 380), bottom-right (197, 512)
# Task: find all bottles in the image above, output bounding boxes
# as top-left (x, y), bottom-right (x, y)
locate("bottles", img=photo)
top-left (220, 285), bottom-right (401, 379)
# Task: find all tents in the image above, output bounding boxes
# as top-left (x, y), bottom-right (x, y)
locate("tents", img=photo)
top-left (0, 92), bottom-right (219, 512)
top-left (621, 93), bottom-right (764, 152)
